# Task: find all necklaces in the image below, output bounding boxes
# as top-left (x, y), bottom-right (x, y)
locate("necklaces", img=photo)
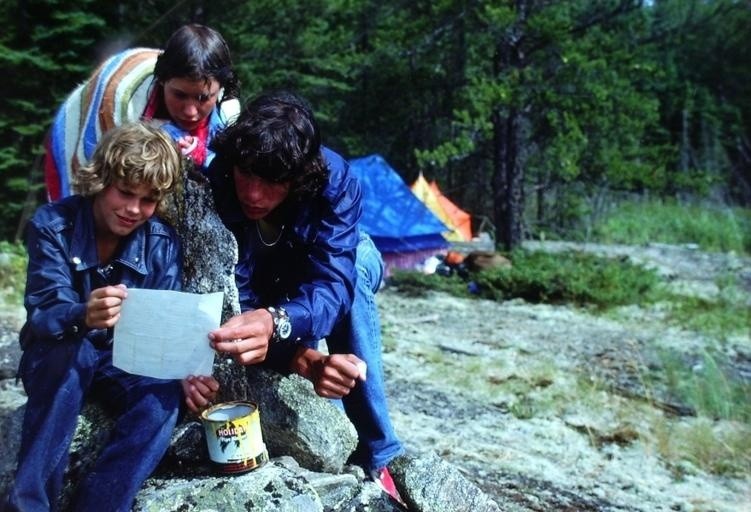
top-left (254, 218), bottom-right (287, 248)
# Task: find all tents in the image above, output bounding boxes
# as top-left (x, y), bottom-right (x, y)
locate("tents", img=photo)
top-left (347, 152), bottom-right (471, 278)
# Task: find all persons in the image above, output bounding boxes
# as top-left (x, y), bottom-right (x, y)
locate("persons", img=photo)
top-left (44, 23), bottom-right (241, 206)
top-left (207, 89), bottom-right (408, 512)
top-left (0, 120), bottom-right (220, 512)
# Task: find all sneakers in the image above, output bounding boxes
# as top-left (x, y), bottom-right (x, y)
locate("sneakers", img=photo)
top-left (368, 462), bottom-right (409, 510)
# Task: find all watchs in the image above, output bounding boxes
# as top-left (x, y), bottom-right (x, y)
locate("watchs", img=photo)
top-left (268, 306), bottom-right (292, 343)
